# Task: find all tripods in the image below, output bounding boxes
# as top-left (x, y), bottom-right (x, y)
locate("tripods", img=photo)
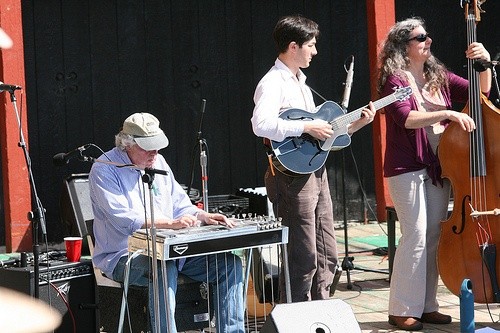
top-left (340, 148), bottom-right (389, 288)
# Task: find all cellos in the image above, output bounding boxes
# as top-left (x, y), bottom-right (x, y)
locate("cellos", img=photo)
top-left (436, 0), bottom-right (500, 304)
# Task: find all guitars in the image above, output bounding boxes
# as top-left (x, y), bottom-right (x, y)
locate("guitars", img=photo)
top-left (263, 85), bottom-right (413, 178)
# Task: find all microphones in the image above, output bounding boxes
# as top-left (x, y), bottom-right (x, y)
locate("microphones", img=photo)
top-left (53, 145), bottom-right (89, 161)
top-left (0, 82), bottom-right (20, 92)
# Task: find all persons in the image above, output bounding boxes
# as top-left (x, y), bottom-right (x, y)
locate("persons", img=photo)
top-left (249, 16), bottom-right (376, 304)
top-left (373, 17), bottom-right (493, 332)
top-left (87, 111), bottom-right (247, 333)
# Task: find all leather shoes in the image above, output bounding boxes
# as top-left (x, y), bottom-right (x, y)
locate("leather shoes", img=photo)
top-left (388, 315), bottom-right (422, 330)
top-left (418, 311), bottom-right (452, 324)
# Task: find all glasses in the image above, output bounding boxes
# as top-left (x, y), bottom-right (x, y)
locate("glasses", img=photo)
top-left (405, 32), bottom-right (430, 43)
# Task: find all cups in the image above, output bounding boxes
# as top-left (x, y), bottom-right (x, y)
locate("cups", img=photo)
top-left (64, 237), bottom-right (83, 262)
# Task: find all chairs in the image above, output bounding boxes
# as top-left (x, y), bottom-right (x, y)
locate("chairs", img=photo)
top-left (84, 219), bottom-right (149, 333)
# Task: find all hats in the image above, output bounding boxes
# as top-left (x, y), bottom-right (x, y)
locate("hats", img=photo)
top-left (123, 112), bottom-right (169, 151)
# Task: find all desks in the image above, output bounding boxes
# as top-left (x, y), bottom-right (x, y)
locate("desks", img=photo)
top-left (386, 204), bottom-right (400, 288)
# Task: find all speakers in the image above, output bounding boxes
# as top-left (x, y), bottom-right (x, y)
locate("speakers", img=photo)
top-left (259, 299), bottom-right (364, 333)
top-left (0, 255), bottom-right (97, 333)
